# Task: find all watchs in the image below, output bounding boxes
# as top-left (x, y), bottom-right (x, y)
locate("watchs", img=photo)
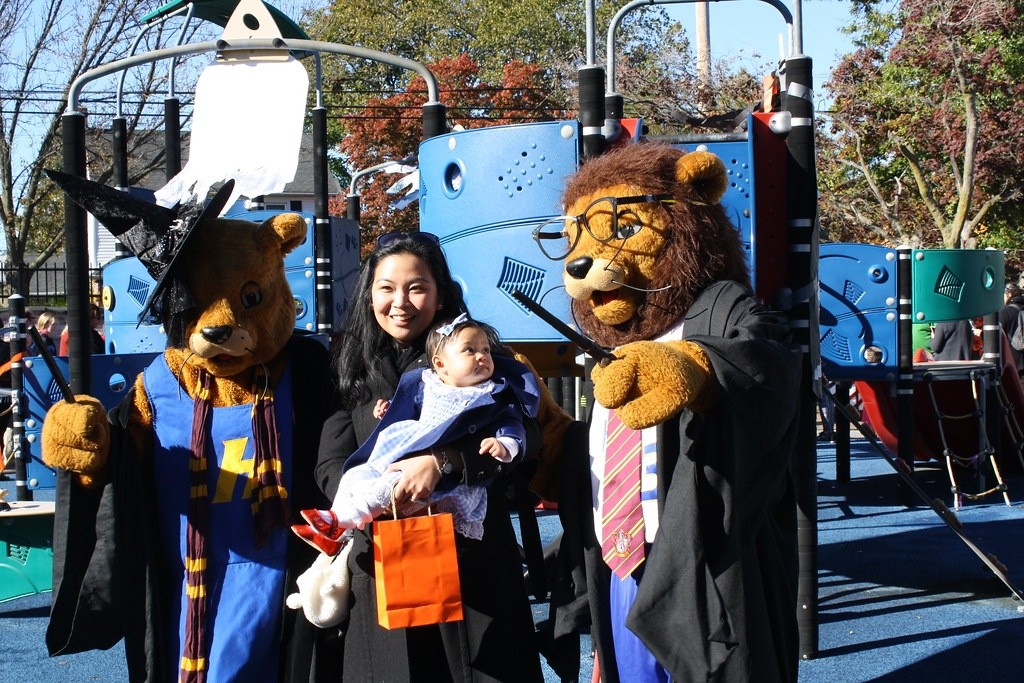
top-left (438, 448), bottom-right (453, 477)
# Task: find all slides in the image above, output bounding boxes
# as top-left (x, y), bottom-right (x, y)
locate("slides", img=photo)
top-left (852, 331), bottom-right (1024, 470)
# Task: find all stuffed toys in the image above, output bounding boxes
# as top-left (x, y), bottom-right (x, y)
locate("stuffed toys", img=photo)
top-left (498, 140), bottom-right (802, 683)
top-left (41, 215), bottom-right (339, 683)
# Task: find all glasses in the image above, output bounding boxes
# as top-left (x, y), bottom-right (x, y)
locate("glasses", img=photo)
top-left (93, 314), bottom-right (100, 320)
top-left (378, 232), bottom-right (439, 246)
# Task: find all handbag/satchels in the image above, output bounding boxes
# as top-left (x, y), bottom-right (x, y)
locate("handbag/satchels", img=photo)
top-left (373, 477), bottom-right (463, 631)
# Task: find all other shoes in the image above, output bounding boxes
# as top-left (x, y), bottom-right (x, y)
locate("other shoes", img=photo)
top-left (291, 509), bottom-right (347, 557)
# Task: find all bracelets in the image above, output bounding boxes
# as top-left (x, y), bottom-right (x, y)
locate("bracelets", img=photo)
top-left (432, 453), bottom-right (444, 478)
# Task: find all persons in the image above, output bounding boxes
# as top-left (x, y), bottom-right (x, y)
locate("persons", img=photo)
top-left (313, 230), bottom-right (546, 683)
top-left (23, 311), bottom-right (57, 358)
top-left (289, 312), bottom-right (527, 558)
top-left (58, 303), bottom-right (104, 358)
top-left (972, 282), bottom-right (1024, 377)
top-left (929, 319), bottom-right (973, 361)
